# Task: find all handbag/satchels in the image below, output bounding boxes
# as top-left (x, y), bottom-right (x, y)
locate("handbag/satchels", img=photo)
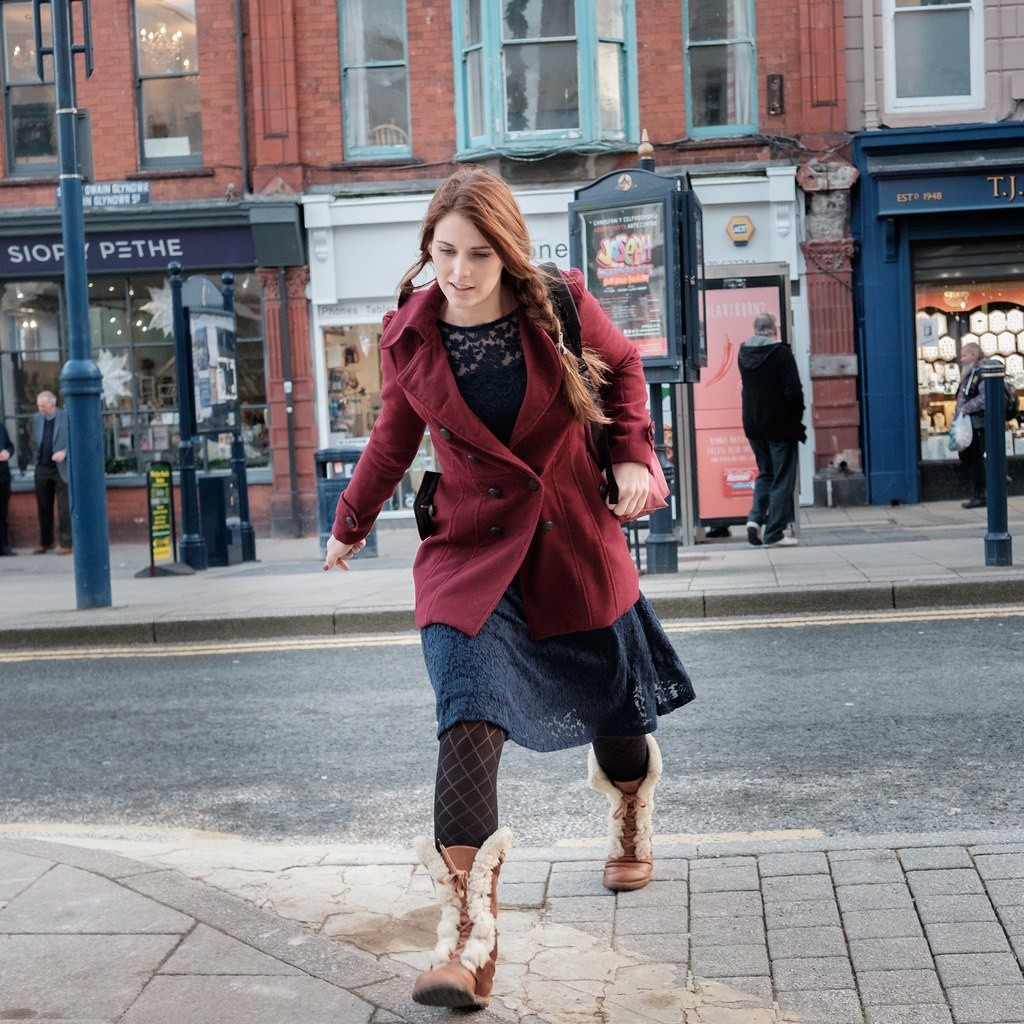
top-left (537, 262), bottom-right (669, 523)
top-left (949, 411), bottom-right (973, 451)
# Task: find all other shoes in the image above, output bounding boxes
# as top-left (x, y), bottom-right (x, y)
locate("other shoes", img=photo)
top-left (1007, 476), bottom-right (1012, 484)
top-left (746, 521), bottom-right (763, 545)
top-left (764, 536), bottom-right (798, 547)
top-left (59, 546), bottom-right (71, 554)
top-left (35, 543), bottom-right (55, 553)
top-left (2, 549), bottom-right (17, 556)
top-left (962, 496), bottom-right (986, 508)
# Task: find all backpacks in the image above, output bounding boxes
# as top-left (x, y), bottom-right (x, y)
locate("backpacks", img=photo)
top-left (966, 365), bottom-right (1019, 421)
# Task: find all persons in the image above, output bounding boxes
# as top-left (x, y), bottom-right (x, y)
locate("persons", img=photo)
top-left (30, 391), bottom-right (72, 555)
top-left (705, 526), bottom-right (730, 536)
top-left (954, 342), bottom-right (985, 508)
top-left (321, 170), bottom-right (697, 1015)
top-left (0, 423), bottom-right (18, 556)
top-left (737, 313), bottom-right (808, 546)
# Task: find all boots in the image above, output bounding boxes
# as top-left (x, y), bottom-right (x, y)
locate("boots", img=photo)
top-left (587, 732), bottom-right (663, 890)
top-left (411, 826), bottom-right (513, 1008)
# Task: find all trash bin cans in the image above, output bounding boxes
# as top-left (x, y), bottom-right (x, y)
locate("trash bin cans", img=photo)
top-left (313, 448), bottom-right (379, 561)
top-left (198, 474), bottom-right (242, 566)
